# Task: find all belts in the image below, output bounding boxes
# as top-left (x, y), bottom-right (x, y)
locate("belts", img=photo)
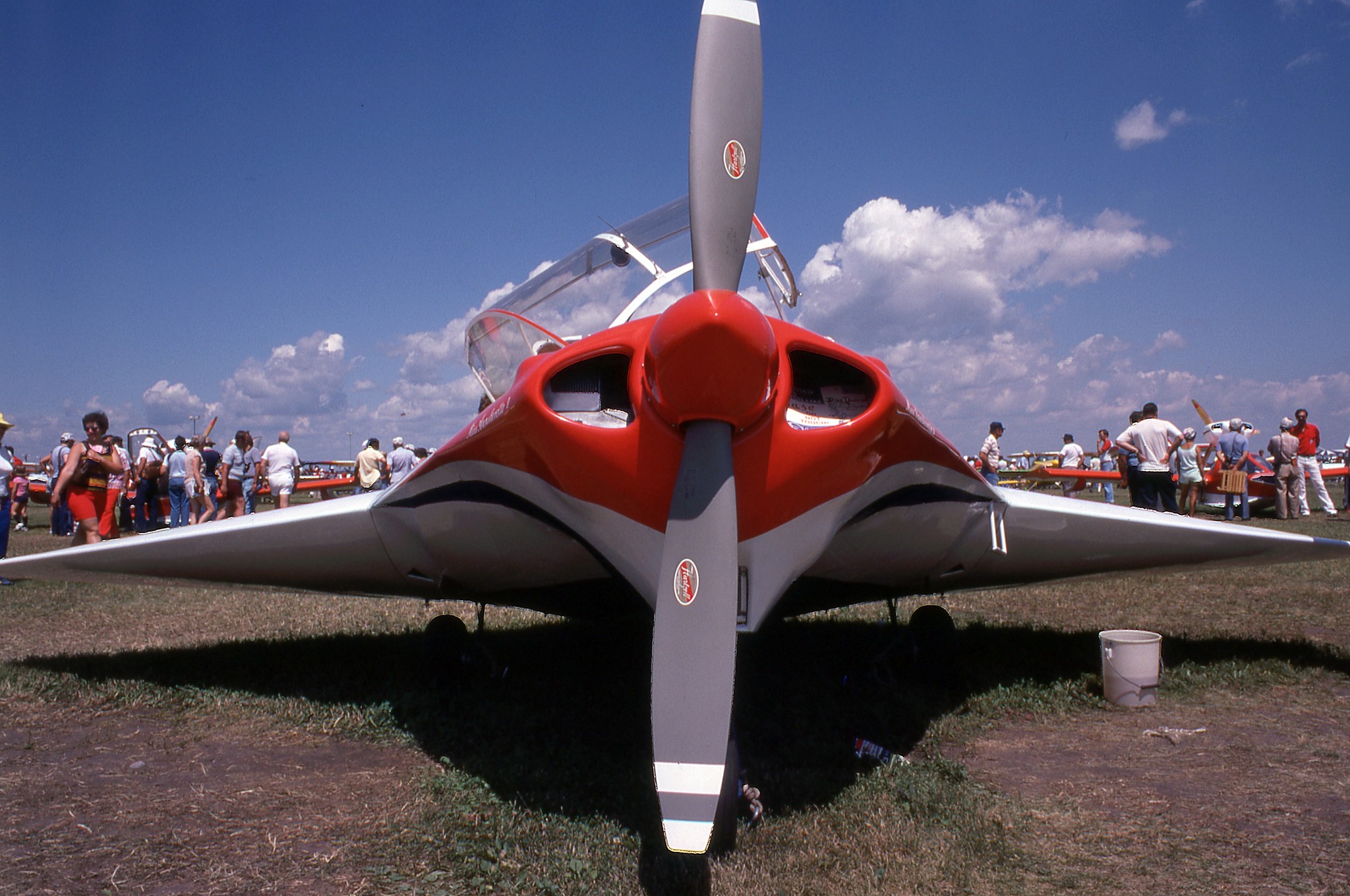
top-left (244, 476), bottom-right (254, 480)
top-left (1298, 454), bottom-right (1315, 458)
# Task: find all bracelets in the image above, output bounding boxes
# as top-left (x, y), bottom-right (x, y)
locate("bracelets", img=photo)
top-left (1136, 450), bottom-right (1140, 455)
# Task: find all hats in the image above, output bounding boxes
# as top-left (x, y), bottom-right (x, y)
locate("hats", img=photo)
top-left (0, 413), bottom-right (15, 429)
top-left (1183, 427), bottom-right (1195, 439)
top-left (141, 437), bottom-right (157, 448)
top-left (1063, 434), bottom-right (1073, 440)
top-left (405, 444), bottom-right (415, 451)
top-left (205, 437), bottom-right (216, 445)
top-left (362, 440), bottom-right (370, 449)
top-left (393, 436), bottom-right (403, 445)
top-left (1023, 450), bottom-right (1030, 455)
top-left (61, 432), bottom-right (76, 443)
top-left (427, 447), bottom-right (437, 453)
top-left (1281, 417), bottom-right (1294, 428)
top-left (990, 422), bottom-right (1006, 429)
top-left (1230, 418), bottom-right (1243, 430)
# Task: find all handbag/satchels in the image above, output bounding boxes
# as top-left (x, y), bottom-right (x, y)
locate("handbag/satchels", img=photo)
top-left (58, 441), bottom-right (92, 486)
top-left (141, 460), bottom-right (163, 479)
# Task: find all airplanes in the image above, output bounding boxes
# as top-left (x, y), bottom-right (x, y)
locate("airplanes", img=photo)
top-left (0, 1), bottom-right (1350, 858)
top-left (0, 412), bottom-right (389, 529)
top-left (975, 399), bottom-right (1350, 515)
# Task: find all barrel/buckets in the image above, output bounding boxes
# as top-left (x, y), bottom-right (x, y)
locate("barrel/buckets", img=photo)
top-left (1098, 630), bottom-right (1163, 707)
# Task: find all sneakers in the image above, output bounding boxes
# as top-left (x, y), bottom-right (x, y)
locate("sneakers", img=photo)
top-left (13, 522), bottom-right (24, 532)
top-left (21, 526), bottom-right (29, 531)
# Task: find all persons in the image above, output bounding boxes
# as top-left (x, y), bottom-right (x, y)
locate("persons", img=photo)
top-left (1259, 450), bottom-right (1264, 456)
top-left (1114, 402), bottom-right (1184, 517)
top-left (1289, 408), bottom-right (1339, 519)
top-left (1117, 411), bottom-right (1145, 507)
top-left (4, 446), bottom-right (24, 507)
top-left (1215, 418), bottom-right (1254, 521)
top-left (979, 421), bottom-right (1006, 485)
top-left (49, 411), bottom-right (126, 549)
top-left (40, 433), bottom-right (434, 541)
top-left (1094, 428), bottom-right (1116, 506)
top-left (1344, 434), bottom-right (1350, 513)
top-left (10, 465), bottom-right (31, 533)
top-left (0, 414), bottom-right (19, 587)
top-left (1057, 432), bottom-right (1085, 499)
top-left (1175, 428), bottom-right (1207, 521)
top-left (962, 446), bottom-right (1118, 496)
top-left (1267, 417), bottom-right (1301, 521)
